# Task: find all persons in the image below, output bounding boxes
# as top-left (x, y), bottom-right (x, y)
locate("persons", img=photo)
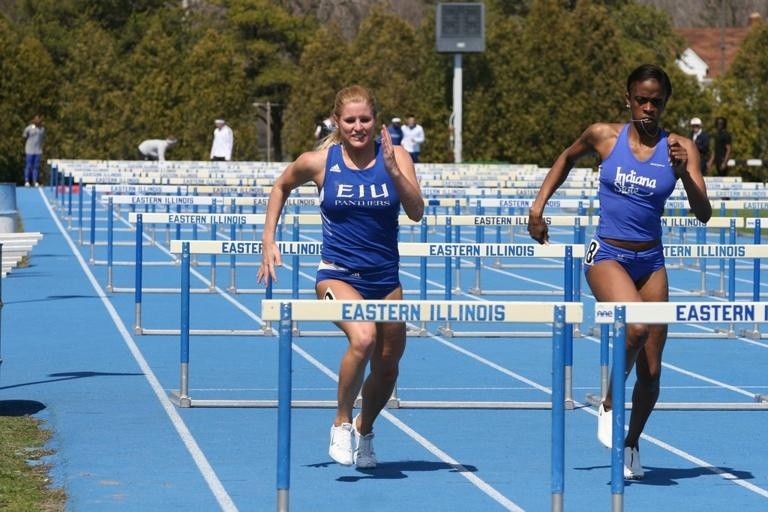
top-left (527, 63), bottom-right (712, 480)
top-left (21, 112), bottom-right (46, 187)
top-left (209, 115), bottom-right (234, 160)
top-left (137, 135), bottom-right (177, 160)
top-left (254, 85), bottom-right (425, 471)
top-left (687, 117), bottom-right (709, 177)
top-left (375, 113), bottom-right (425, 163)
top-left (313, 110), bottom-right (336, 141)
top-left (706, 116), bottom-right (732, 200)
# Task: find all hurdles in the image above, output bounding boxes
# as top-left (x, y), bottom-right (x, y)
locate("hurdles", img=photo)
top-left (47, 159), bottom-right (768, 512)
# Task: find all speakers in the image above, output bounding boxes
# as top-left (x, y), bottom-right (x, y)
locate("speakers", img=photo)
top-left (436, 3), bottom-right (485, 53)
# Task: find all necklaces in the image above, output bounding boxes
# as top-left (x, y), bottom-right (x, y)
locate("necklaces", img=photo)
top-left (631, 115), bottom-right (661, 140)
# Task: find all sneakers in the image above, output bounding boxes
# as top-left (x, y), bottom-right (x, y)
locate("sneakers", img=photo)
top-left (353, 413), bottom-right (376, 467)
top-left (597, 403), bottom-right (613, 448)
top-left (623, 444), bottom-right (644, 480)
top-left (329, 422), bottom-right (354, 466)
